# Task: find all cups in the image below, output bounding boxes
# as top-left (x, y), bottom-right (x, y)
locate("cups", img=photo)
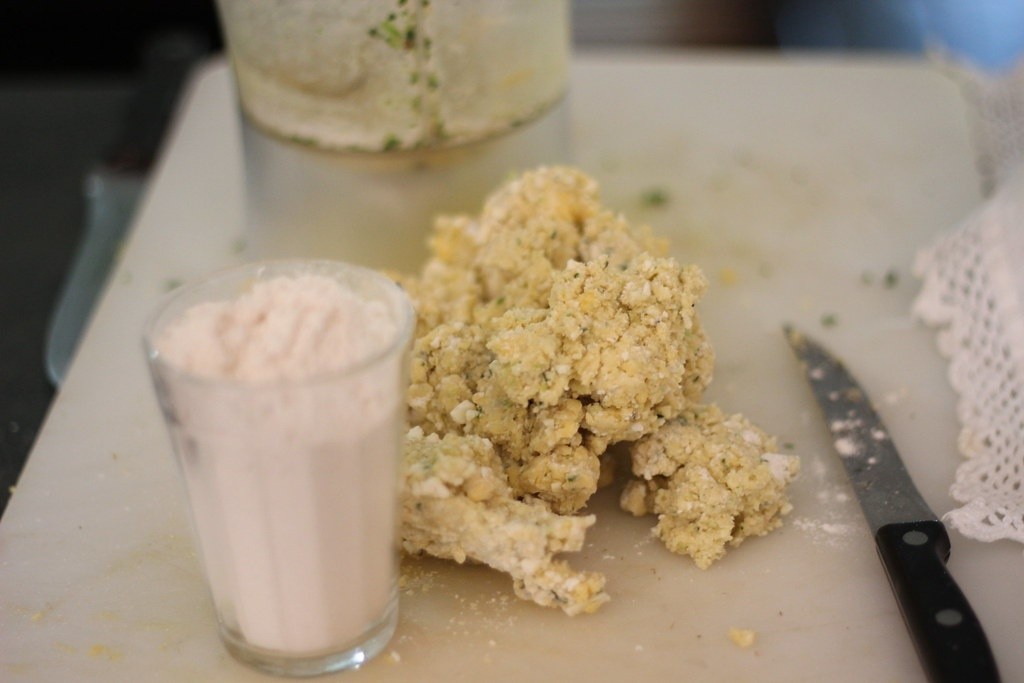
top-left (217, 0), bottom-right (573, 154)
top-left (138, 260), bottom-right (419, 681)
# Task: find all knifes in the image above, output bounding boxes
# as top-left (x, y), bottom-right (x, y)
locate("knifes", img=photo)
top-left (779, 322), bottom-right (1004, 683)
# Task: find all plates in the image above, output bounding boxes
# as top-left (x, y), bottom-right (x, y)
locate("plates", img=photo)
top-left (0, 52), bottom-right (1024, 682)
